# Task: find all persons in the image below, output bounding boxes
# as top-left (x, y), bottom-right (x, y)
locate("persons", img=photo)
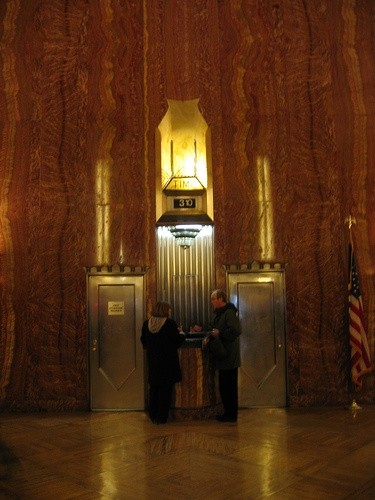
top-left (140, 301), bottom-right (187, 425)
top-left (202, 289), bottom-right (241, 424)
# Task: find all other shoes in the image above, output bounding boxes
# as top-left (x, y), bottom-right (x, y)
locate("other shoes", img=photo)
top-left (216, 415), bottom-right (237, 425)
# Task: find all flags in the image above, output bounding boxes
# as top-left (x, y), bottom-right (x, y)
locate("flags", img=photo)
top-left (348, 238), bottom-right (374, 394)
